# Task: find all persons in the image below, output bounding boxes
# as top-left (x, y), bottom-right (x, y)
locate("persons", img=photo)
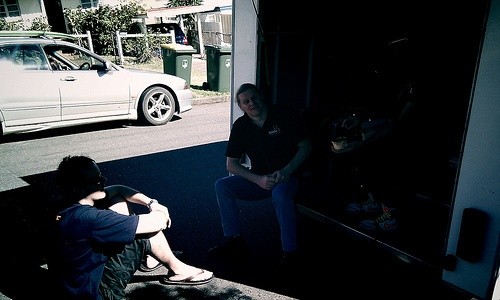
top-left (46, 155), bottom-right (213, 300)
top-left (207, 82), bottom-right (312, 275)
top-left (341, 27), bottom-right (451, 233)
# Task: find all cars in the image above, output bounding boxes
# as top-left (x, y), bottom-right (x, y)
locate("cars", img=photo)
top-left (0, 31), bottom-right (79, 43)
top-left (0, 39), bottom-right (194, 136)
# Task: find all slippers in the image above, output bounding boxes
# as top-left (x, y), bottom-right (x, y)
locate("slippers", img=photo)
top-left (163, 265), bottom-right (214, 285)
top-left (140, 261), bottom-right (164, 273)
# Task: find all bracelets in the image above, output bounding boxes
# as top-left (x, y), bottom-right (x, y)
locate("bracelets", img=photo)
top-left (146, 199), bottom-right (158, 210)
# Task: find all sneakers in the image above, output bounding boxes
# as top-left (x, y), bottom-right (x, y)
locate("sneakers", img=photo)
top-left (347, 192), bottom-right (381, 212)
top-left (361, 203), bottom-right (397, 231)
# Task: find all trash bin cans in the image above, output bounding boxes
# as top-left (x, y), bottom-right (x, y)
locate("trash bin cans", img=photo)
top-left (203, 42), bottom-right (232, 93)
top-left (160, 43), bottom-right (197, 87)
